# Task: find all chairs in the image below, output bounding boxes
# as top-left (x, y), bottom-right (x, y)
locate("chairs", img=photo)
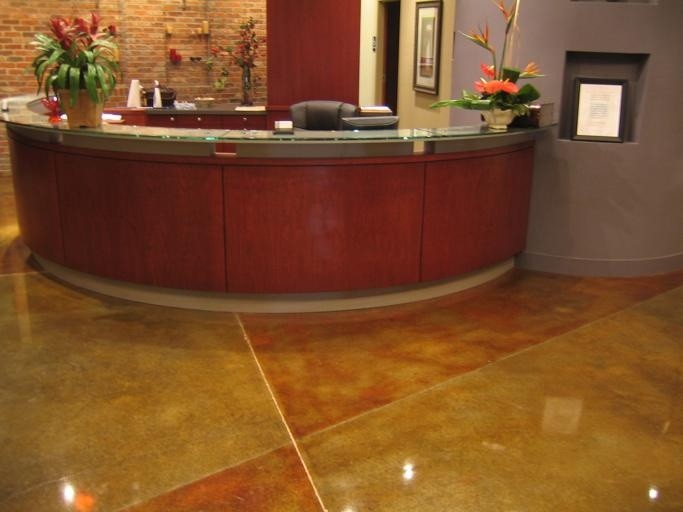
top-left (289, 100), bottom-right (358, 130)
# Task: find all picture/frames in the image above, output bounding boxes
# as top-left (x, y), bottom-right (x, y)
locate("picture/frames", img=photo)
top-left (412, 0), bottom-right (443, 96)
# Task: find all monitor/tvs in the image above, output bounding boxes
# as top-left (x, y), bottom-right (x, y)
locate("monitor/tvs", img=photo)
top-left (340, 116), bottom-right (399, 131)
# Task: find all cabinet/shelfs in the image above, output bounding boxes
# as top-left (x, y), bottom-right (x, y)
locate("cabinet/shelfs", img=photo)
top-left (109, 107), bottom-right (275, 130)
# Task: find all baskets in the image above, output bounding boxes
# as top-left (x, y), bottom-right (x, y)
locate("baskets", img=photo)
top-left (144, 83), bottom-right (177, 107)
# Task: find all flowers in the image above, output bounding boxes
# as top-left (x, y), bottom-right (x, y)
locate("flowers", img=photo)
top-left (29, 12), bottom-right (124, 112)
top-left (425, 0), bottom-right (547, 115)
top-left (211, 15), bottom-right (268, 69)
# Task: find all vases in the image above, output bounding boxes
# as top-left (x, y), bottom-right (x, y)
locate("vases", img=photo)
top-left (480, 110), bottom-right (519, 132)
top-left (56, 88), bottom-right (105, 129)
top-left (241, 67), bottom-right (253, 104)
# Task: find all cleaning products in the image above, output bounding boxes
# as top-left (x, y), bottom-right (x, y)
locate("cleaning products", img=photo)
top-left (154, 79), bottom-right (164, 107)
